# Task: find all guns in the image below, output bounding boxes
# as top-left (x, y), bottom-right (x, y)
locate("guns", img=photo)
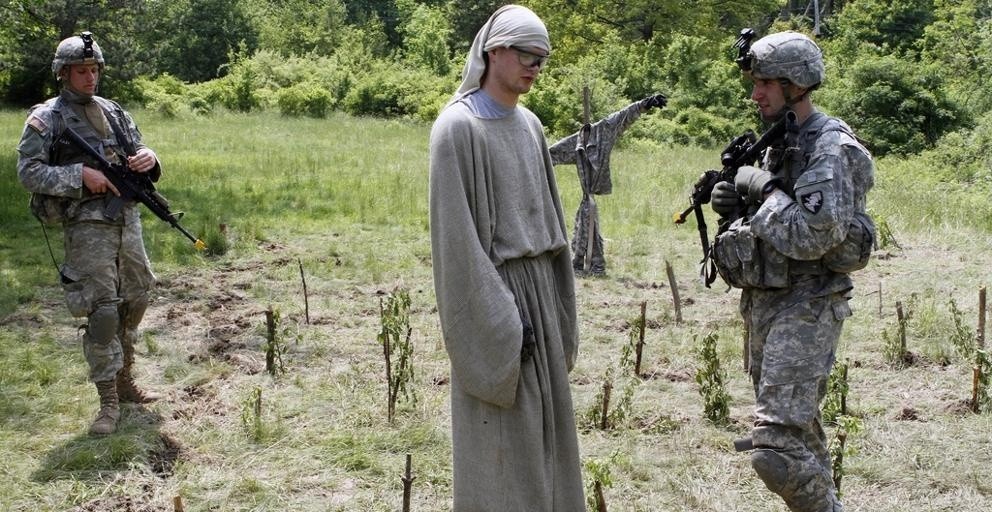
top-left (673, 110), bottom-right (801, 224)
top-left (48, 126), bottom-right (204, 249)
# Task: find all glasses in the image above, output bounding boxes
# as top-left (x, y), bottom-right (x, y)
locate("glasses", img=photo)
top-left (506, 44), bottom-right (551, 72)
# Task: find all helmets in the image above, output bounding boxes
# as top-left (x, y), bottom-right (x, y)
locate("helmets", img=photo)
top-left (51, 35), bottom-right (105, 73)
top-left (739, 30), bottom-right (825, 91)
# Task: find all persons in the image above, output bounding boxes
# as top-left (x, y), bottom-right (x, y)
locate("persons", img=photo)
top-left (14, 31), bottom-right (164, 443)
top-left (424, 0), bottom-right (590, 512)
top-left (706, 26), bottom-right (880, 512)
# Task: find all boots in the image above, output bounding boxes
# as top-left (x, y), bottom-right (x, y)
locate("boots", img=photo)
top-left (116, 343), bottom-right (163, 404)
top-left (88, 379), bottom-right (122, 438)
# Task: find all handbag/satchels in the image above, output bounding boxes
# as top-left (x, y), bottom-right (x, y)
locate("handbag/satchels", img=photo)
top-left (30, 190), bottom-right (79, 226)
top-left (711, 215), bottom-right (791, 289)
top-left (823, 211), bottom-right (877, 273)
